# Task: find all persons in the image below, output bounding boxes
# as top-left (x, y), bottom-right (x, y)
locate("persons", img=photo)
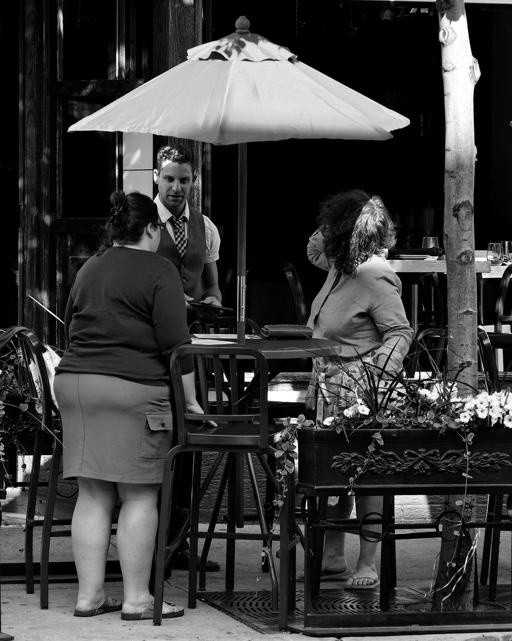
top-left (294, 188), bottom-right (417, 593)
top-left (148, 140), bottom-right (223, 583)
top-left (51, 186), bottom-right (221, 623)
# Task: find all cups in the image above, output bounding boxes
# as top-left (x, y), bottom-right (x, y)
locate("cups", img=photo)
top-left (486, 243), bottom-right (504, 266)
top-left (421, 237), bottom-right (440, 250)
top-left (499, 241), bottom-right (512, 266)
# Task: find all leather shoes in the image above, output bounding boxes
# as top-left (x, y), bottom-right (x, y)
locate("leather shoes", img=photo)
top-left (171, 548), bottom-right (221, 571)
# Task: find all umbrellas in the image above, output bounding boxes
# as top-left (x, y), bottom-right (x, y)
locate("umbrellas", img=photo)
top-left (64, 12), bottom-right (416, 532)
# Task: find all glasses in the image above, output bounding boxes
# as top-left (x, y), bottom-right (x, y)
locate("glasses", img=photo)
top-left (154, 223), bottom-right (166, 231)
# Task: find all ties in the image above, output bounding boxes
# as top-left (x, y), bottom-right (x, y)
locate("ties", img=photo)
top-left (170, 218), bottom-right (186, 256)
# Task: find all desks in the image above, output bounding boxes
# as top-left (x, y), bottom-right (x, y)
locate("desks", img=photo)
top-left (383, 249), bottom-right (492, 332)
top-left (161, 333), bottom-right (343, 594)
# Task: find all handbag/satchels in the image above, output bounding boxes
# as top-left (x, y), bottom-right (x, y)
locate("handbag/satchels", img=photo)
top-left (0, 325), bottom-right (60, 417)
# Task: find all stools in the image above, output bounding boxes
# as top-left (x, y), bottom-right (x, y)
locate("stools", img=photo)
top-left (11, 327), bottom-right (122, 610)
top-left (149, 343), bottom-right (298, 630)
top-left (189, 315), bottom-right (278, 577)
top-left (475, 329), bottom-right (511, 606)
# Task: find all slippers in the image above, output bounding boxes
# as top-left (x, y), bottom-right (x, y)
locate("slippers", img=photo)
top-left (121, 595), bottom-right (184, 620)
top-left (296, 563), bottom-right (352, 582)
top-left (74, 595), bottom-right (122, 616)
top-left (343, 570), bottom-right (380, 590)
